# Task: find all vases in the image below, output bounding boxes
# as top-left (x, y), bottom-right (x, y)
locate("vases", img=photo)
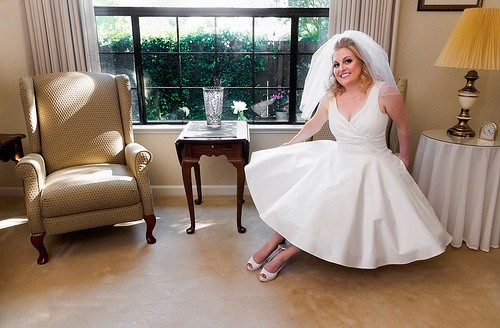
top-left (275, 111), bottom-right (289, 121)
top-left (296, 112), bottom-right (306, 122)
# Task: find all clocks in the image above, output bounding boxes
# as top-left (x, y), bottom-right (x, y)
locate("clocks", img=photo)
top-left (478, 119), bottom-right (498, 142)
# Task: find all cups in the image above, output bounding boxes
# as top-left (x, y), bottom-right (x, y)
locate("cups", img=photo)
top-left (202, 85), bottom-right (224, 129)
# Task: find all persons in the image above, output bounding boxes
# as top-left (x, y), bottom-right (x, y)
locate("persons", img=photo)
top-left (245, 30), bottom-right (453, 283)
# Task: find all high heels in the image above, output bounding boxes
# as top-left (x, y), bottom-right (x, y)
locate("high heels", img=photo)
top-left (247, 244), bottom-right (279, 271)
top-left (259, 247), bottom-right (288, 282)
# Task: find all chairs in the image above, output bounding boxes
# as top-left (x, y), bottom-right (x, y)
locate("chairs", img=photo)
top-left (15, 71), bottom-right (158, 265)
top-left (304, 77), bottom-right (408, 152)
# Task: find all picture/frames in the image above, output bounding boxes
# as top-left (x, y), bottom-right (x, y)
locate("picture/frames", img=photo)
top-left (416, 0), bottom-right (484, 12)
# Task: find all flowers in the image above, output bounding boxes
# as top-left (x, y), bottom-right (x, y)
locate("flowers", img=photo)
top-left (231, 100), bottom-right (249, 122)
top-left (263, 90), bottom-right (288, 112)
top-left (178, 106), bottom-right (191, 120)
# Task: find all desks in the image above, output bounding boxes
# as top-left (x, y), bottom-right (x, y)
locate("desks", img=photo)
top-left (410, 127), bottom-right (500, 254)
top-left (173, 120), bottom-right (252, 233)
top-left (0, 134), bottom-right (27, 165)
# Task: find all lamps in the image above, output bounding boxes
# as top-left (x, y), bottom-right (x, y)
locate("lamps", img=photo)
top-left (434, 7), bottom-right (500, 138)
